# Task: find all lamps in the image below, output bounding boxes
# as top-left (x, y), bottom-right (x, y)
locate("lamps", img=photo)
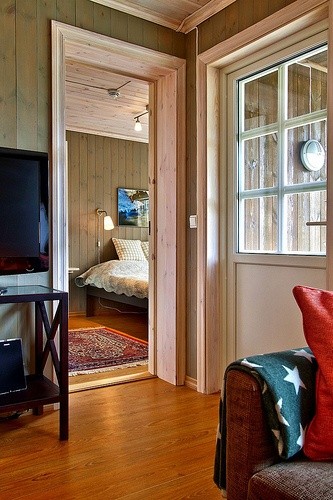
top-left (97, 208), bottom-right (115, 231)
top-left (134, 115), bottom-right (142, 132)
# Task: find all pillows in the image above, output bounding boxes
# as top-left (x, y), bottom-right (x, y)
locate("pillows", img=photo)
top-left (292, 285), bottom-right (333, 462)
top-left (111, 237), bottom-right (147, 262)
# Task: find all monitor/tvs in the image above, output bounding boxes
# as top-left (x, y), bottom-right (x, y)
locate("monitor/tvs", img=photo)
top-left (0, 147), bottom-right (50, 276)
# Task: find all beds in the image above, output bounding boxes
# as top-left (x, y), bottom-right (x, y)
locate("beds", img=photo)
top-left (77, 257), bottom-right (148, 320)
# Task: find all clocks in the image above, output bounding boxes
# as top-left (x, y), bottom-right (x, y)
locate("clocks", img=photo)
top-left (301, 138), bottom-right (325, 173)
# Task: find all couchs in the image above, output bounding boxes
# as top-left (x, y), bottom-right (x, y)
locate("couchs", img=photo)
top-left (214, 347), bottom-right (333, 500)
